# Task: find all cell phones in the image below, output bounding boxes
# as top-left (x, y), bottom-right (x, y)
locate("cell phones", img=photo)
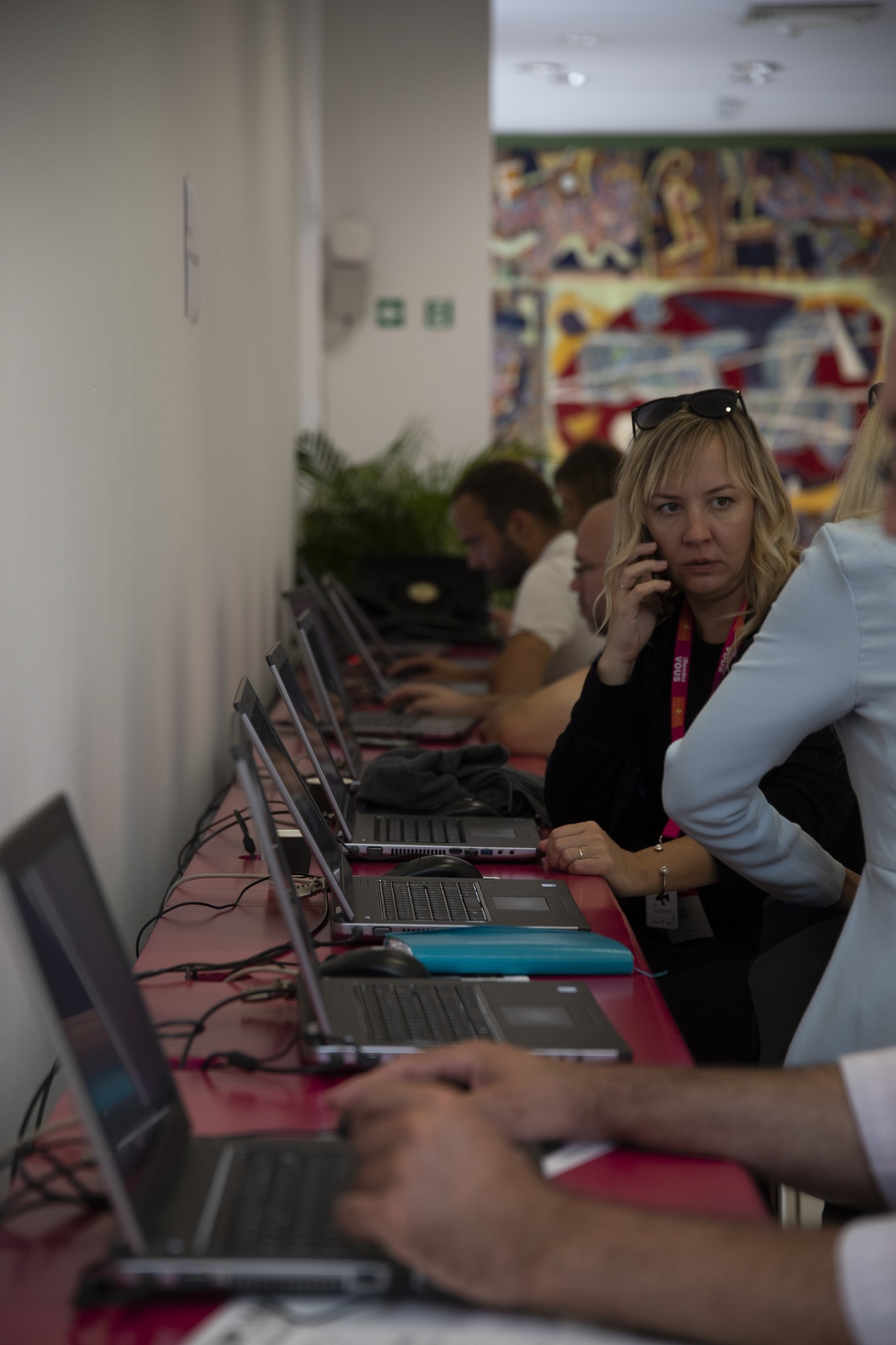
top-left (637, 525), bottom-right (672, 613)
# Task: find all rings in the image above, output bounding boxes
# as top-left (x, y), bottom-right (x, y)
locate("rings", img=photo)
top-left (578, 847), bottom-right (583, 859)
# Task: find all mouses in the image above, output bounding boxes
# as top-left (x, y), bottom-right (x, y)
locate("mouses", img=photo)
top-left (318, 946), bottom-right (431, 979)
top-left (381, 855), bottom-right (484, 879)
top-left (392, 664), bottom-right (432, 680)
top-left (390, 696), bottom-right (419, 713)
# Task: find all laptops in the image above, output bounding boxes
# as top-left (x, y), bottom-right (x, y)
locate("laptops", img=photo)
top-left (265, 640), bottom-right (546, 861)
top-left (318, 573), bottom-right (491, 702)
top-left (233, 676), bottom-right (593, 944)
top-left (310, 602), bottom-right (482, 740)
top-left (232, 714), bottom-right (635, 1075)
top-left (1, 795), bottom-right (434, 1290)
top-left (332, 575), bottom-right (489, 673)
top-left (296, 607), bottom-right (367, 790)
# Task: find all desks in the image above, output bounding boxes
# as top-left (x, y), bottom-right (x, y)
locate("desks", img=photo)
top-left (0, 646), bottom-right (775, 1345)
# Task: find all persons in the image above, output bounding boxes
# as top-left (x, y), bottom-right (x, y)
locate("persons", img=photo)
top-left (553, 438), bottom-right (629, 533)
top-left (662, 299), bottom-right (896, 1229)
top-left (537, 385), bottom-right (867, 1230)
top-left (316, 1040), bottom-right (896, 1345)
top-left (389, 456), bottom-right (608, 699)
top-left (383, 497), bottom-right (621, 754)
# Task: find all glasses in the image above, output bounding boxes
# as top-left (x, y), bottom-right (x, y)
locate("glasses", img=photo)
top-left (573, 562), bottom-right (606, 578)
top-left (630, 389), bottom-right (750, 443)
top-left (868, 381), bottom-right (888, 410)
